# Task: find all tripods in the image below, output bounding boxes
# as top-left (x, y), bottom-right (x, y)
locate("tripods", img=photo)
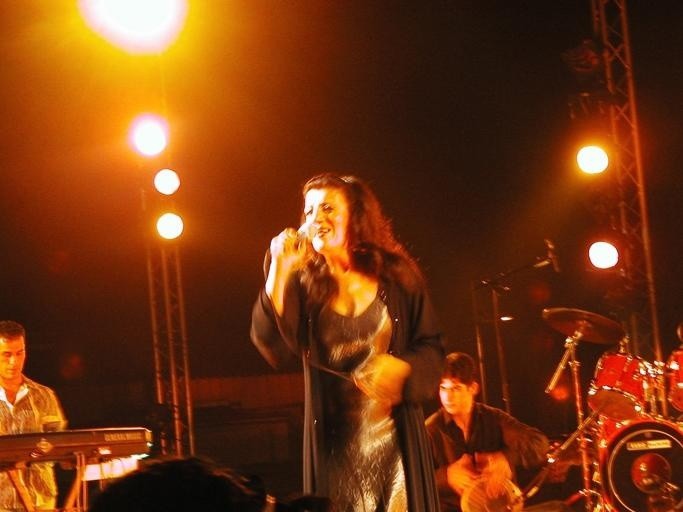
top-left (545, 330), bottom-right (619, 512)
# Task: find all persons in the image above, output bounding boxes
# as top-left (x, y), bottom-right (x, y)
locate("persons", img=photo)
top-left (250, 170), bottom-right (449, 512)
top-left (423, 351), bottom-right (550, 511)
top-left (0, 319), bottom-right (102, 512)
top-left (87, 456), bottom-right (261, 511)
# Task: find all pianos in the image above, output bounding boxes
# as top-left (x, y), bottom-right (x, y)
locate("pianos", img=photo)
top-left (1, 428), bottom-right (153, 464)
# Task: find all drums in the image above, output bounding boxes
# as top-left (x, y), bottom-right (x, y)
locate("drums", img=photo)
top-left (461, 478), bottom-right (524, 512)
top-left (596, 413), bottom-right (683, 512)
top-left (587, 352), bottom-right (652, 420)
top-left (666, 351), bottom-right (683, 411)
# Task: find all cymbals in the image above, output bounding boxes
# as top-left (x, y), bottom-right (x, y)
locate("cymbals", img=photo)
top-left (541, 307), bottom-right (626, 344)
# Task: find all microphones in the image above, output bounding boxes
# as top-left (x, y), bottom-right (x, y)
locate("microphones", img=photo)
top-left (294, 222), bottom-right (316, 251)
top-left (544, 238), bottom-right (561, 273)
